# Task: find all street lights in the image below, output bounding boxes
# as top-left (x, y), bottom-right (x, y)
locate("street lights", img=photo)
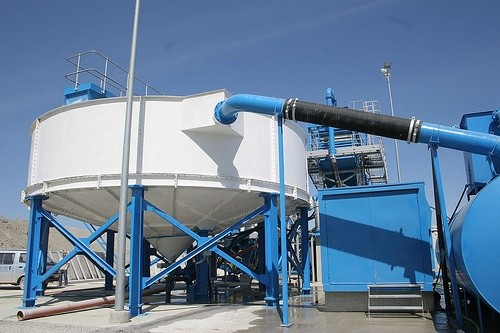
top-left (380, 59), bottom-right (401, 184)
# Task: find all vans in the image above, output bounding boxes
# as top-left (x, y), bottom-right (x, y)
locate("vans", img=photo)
top-left (0, 250), bottom-right (62, 291)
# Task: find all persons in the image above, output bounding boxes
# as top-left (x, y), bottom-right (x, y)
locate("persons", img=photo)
top-left (58, 253), bottom-right (70, 286)
